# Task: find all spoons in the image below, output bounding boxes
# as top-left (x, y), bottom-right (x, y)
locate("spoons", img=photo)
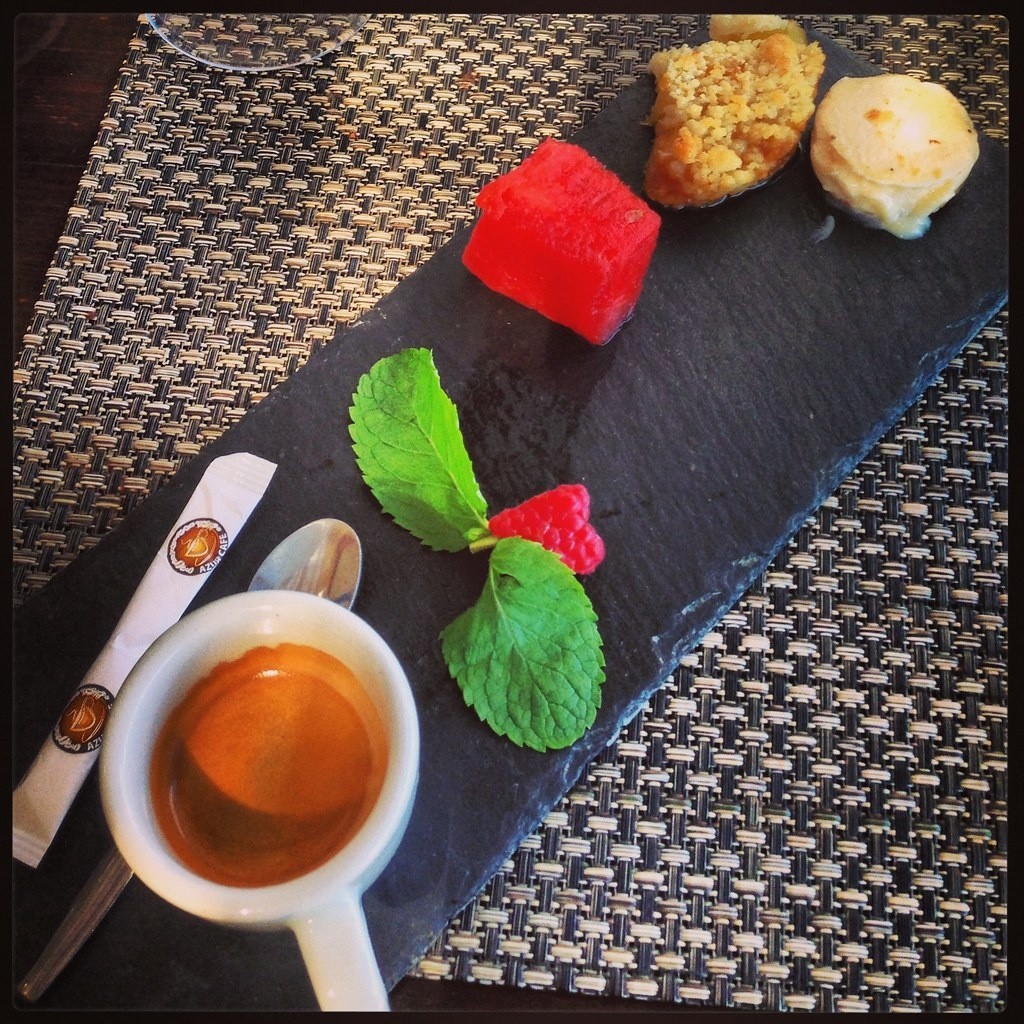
top-left (17, 517), bottom-right (363, 1002)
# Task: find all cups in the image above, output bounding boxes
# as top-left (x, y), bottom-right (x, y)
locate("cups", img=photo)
top-left (97, 590), bottom-right (423, 1012)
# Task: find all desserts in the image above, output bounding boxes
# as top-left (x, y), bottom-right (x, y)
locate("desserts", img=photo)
top-left (810, 73), bottom-right (980, 238)
top-left (641, 13), bottom-right (828, 209)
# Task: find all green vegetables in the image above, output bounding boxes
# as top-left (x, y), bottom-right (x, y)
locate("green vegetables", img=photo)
top-left (347, 346), bottom-right (606, 752)
